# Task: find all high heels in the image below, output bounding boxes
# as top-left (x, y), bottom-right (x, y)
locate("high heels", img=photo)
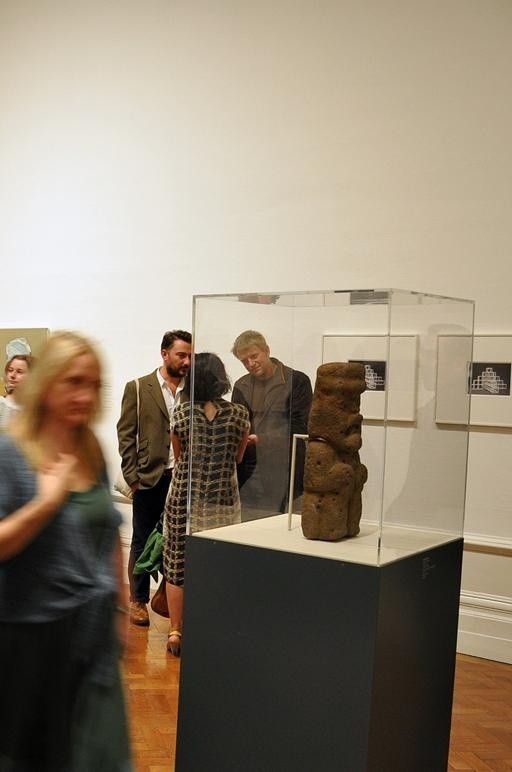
top-left (166, 630), bottom-right (181, 656)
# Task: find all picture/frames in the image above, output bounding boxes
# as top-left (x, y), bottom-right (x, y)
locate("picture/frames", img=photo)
top-left (322, 334), bottom-right (418, 421)
top-left (434, 334), bottom-right (512, 427)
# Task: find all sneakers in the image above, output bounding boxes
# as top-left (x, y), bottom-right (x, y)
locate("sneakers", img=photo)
top-left (129, 599), bottom-right (151, 627)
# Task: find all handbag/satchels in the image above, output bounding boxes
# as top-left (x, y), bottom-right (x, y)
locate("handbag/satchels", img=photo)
top-left (150, 578), bottom-right (169, 621)
top-left (113, 476), bottom-right (136, 498)
top-left (132, 513), bottom-right (165, 576)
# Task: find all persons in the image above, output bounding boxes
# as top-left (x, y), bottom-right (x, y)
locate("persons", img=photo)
top-left (0, 330), bottom-right (130, 772)
top-left (162, 353), bottom-right (252, 656)
top-left (0, 354), bottom-right (37, 429)
top-left (117, 329), bottom-right (192, 627)
top-left (230, 330), bottom-right (313, 523)
